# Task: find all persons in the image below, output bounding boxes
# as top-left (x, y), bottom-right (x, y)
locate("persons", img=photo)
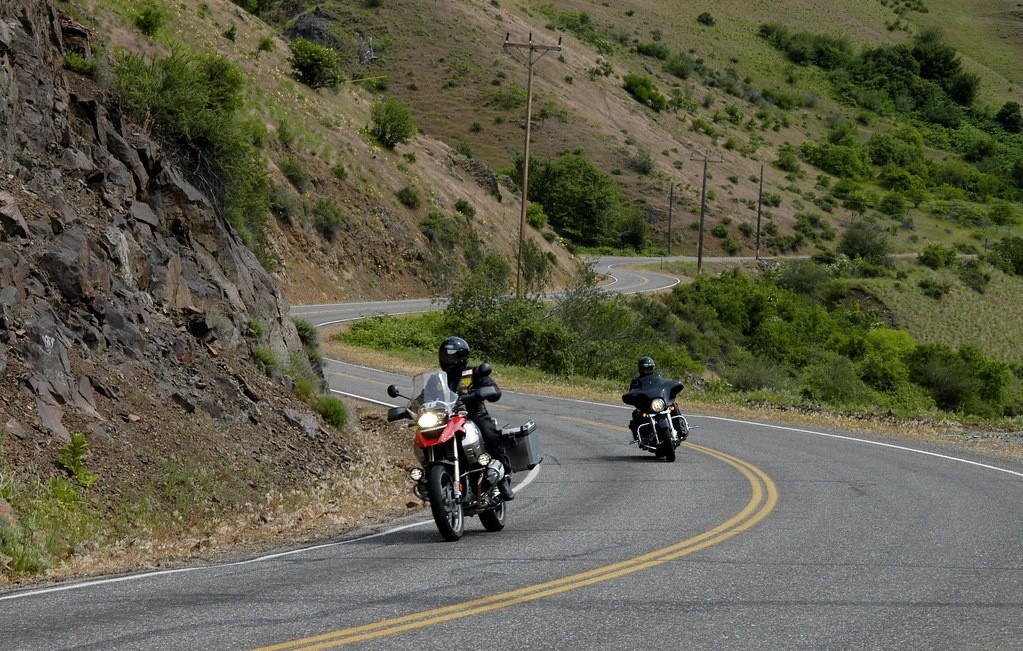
top-left (628, 357), bottom-right (688, 440)
top-left (407, 336), bottom-right (515, 501)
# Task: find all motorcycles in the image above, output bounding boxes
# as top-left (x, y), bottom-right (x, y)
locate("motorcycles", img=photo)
top-left (387, 361), bottom-right (544, 542)
top-left (621, 372), bottom-right (699, 462)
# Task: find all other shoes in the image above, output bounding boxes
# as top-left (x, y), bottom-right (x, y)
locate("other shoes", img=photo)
top-left (677, 430), bottom-right (687, 437)
top-left (498, 477), bottom-right (515, 501)
top-left (639, 441), bottom-right (647, 448)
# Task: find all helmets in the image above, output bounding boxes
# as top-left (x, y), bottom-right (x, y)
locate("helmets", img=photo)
top-left (439, 336), bottom-right (470, 372)
top-left (638, 357), bottom-right (655, 375)
